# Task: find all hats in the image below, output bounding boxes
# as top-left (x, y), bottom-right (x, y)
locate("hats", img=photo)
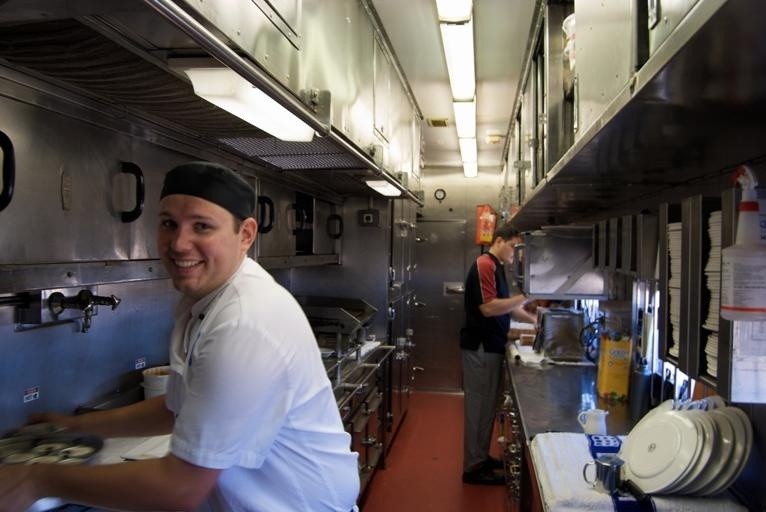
top-left (158, 160), bottom-right (257, 221)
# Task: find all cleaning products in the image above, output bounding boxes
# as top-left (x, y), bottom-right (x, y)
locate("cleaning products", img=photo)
top-left (715, 163), bottom-right (766, 320)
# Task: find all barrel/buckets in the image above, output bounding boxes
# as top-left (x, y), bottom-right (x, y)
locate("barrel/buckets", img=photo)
top-left (139, 363), bottom-right (173, 402)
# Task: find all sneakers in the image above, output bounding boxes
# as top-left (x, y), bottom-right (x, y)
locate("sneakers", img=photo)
top-left (488, 456), bottom-right (504, 469)
top-left (462, 469), bottom-right (506, 486)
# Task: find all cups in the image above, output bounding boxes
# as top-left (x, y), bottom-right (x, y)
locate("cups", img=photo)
top-left (584, 452), bottom-right (625, 494)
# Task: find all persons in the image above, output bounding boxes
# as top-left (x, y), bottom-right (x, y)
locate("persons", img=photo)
top-left (455, 222), bottom-right (541, 487)
top-left (0, 158), bottom-right (360, 511)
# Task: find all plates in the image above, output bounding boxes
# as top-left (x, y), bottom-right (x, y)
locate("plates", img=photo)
top-left (613, 392), bottom-right (756, 502)
top-left (664, 217), bottom-right (690, 362)
top-left (703, 208), bottom-right (725, 383)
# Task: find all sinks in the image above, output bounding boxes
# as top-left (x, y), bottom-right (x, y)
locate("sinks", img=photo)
top-left (76, 386), bottom-right (145, 411)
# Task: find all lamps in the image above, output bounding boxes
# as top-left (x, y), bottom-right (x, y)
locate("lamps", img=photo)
top-left (435, 0), bottom-right (478, 177)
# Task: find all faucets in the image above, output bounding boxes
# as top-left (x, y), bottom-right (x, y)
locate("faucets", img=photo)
top-left (48, 289), bottom-right (122, 333)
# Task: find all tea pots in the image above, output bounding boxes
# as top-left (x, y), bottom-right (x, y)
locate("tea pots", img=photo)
top-left (577, 404), bottom-right (613, 439)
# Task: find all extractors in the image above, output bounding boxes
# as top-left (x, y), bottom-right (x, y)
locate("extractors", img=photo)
top-left (0, 1), bottom-right (410, 201)
